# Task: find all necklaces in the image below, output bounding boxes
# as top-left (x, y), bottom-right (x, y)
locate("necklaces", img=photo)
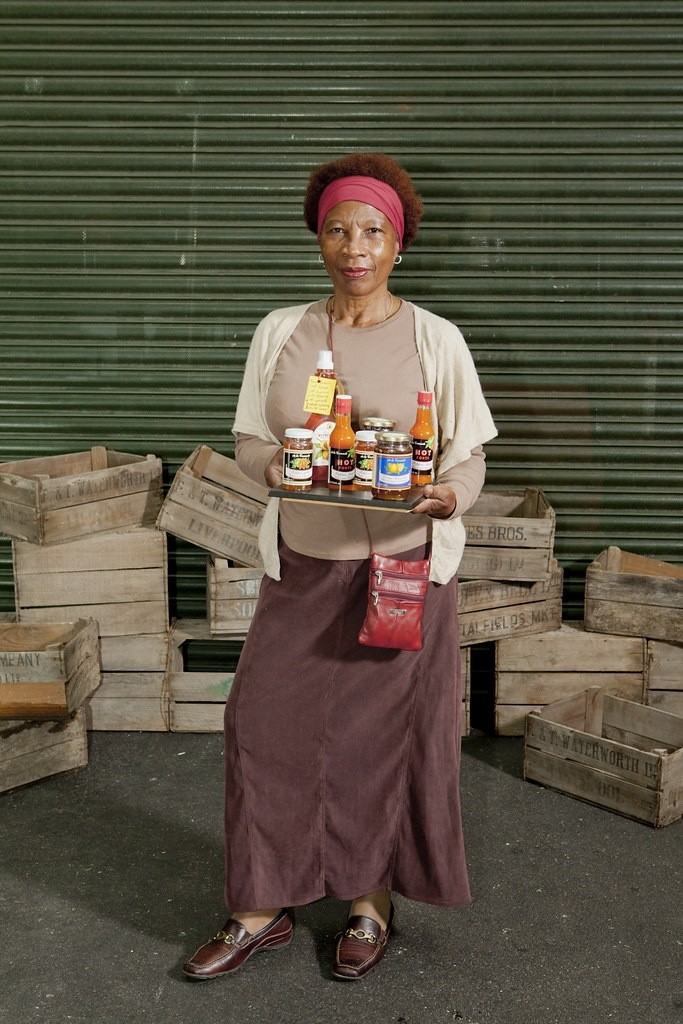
top-left (330, 290), bottom-right (394, 327)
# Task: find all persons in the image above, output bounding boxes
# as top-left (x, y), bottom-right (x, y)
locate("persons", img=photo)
top-left (181, 154), bottom-right (499, 981)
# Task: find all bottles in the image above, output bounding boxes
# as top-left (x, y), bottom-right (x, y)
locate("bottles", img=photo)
top-left (282, 427), bottom-right (314, 492)
top-left (304, 350), bottom-right (336, 481)
top-left (328, 395), bottom-right (357, 492)
top-left (360, 417), bottom-right (396, 435)
top-left (409, 390), bottom-right (436, 486)
top-left (353, 429), bottom-right (382, 488)
top-left (372, 430), bottom-right (413, 502)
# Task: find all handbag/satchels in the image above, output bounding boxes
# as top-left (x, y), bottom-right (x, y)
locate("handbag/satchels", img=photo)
top-left (358, 553), bottom-right (429, 651)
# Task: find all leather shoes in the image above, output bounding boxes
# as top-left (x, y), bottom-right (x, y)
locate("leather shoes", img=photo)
top-left (332, 901), bottom-right (394, 980)
top-left (181, 908), bottom-right (293, 980)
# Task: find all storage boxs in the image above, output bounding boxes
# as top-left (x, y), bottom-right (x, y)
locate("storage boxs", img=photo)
top-left (0, 446), bottom-right (683, 829)
